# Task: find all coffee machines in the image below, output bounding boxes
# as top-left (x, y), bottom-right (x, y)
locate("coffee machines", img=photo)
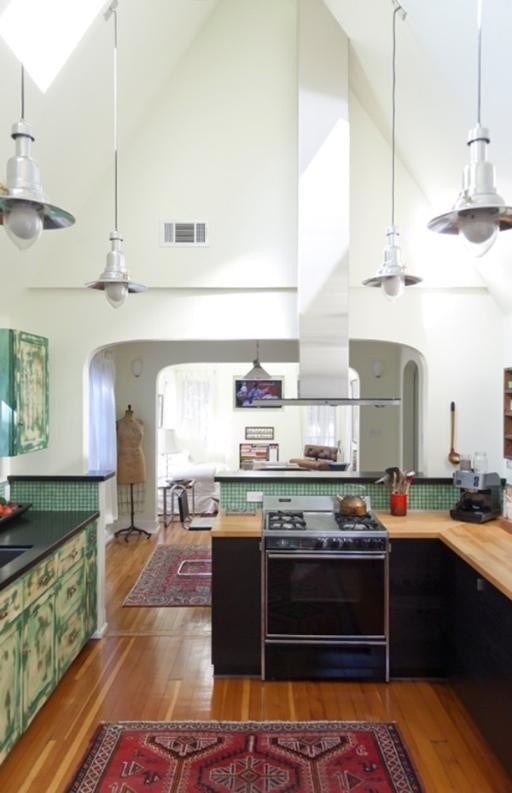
top-left (447, 469), bottom-right (502, 523)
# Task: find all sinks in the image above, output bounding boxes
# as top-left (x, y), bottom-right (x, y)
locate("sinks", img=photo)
top-left (0, 544), bottom-right (35, 572)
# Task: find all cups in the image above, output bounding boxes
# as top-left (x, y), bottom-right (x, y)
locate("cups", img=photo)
top-left (390, 492), bottom-right (408, 516)
top-left (457, 450), bottom-right (472, 471)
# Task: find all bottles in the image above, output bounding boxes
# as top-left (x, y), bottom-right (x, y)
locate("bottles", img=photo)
top-left (470, 450), bottom-right (489, 472)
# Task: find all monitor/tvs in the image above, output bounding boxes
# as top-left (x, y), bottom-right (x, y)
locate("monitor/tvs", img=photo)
top-left (233, 375), bottom-right (286, 412)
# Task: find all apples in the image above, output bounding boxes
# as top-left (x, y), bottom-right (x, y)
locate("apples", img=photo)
top-left (0, 505), bottom-right (20, 516)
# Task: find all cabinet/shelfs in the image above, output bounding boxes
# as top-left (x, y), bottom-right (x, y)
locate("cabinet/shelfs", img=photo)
top-left (0, 522), bottom-right (98, 767)
top-left (503, 367), bottom-right (512, 459)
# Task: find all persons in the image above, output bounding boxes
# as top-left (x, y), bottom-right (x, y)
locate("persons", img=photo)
top-left (116, 410), bottom-right (145, 486)
top-left (248, 384), bottom-right (264, 404)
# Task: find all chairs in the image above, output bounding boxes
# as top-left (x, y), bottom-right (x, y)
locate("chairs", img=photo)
top-left (172, 483), bottom-right (215, 578)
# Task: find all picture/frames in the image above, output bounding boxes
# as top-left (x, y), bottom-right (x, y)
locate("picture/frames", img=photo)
top-left (8, 330), bottom-right (50, 457)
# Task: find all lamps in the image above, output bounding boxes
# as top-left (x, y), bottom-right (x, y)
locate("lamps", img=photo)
top-left (244, 339), bottom-right (271, 380)
top-left (429, 0), bottom-right (511, 242)
top-left (159, 429), bottom-right (182, 481)
top-left (0, 64), bottom-right (76, 252)
top-left (83, 4), bottom-right (147, 306)
top-left (363, 2), bottom-right (421, 295)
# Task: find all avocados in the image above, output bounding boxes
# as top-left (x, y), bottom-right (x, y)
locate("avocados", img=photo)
top-left (0, 497), bottom-right (6, 505)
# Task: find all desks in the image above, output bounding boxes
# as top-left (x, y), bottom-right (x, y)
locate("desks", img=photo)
top-left (157, 479), bottom-right (195, 527)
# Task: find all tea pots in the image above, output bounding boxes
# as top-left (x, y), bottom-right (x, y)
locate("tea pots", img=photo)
top-left (335, 483), bottom-right (370, 518)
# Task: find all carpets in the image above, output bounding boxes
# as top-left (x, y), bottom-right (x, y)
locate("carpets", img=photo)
top-left (122, 543), bottom-right (211, 608)
top-left (64, 719), bottom-right (422, 793)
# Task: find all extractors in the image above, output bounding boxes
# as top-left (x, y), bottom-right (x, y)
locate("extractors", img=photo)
top-left (235, 1), bottom-right (403, 409)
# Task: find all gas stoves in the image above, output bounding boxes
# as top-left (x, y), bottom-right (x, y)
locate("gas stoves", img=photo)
top-left (262, 510), bottom-right (389, 554)
